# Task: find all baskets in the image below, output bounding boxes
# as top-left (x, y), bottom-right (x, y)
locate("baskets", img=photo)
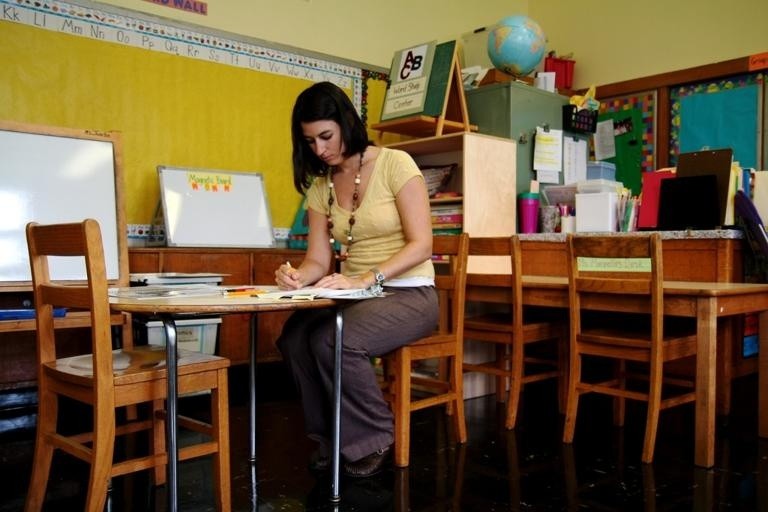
top-left (563, 103), bottom-right (599, 134)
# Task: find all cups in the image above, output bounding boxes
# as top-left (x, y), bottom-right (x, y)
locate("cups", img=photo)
top-left (559, 214), bottom-right (575, 233)
top-left (520, 192), bottom-right (540, 232)
top-left (538, 205), bottom-right (560, 234)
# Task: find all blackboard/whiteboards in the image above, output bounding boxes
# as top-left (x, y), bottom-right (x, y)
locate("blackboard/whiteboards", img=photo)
top-left (157, 165), bottom-right (278, 248)
top-left (0, 121), bottom-right (130, 287)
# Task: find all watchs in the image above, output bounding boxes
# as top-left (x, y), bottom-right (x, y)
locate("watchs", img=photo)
top-left (368, 267), bottom-right (387, 289)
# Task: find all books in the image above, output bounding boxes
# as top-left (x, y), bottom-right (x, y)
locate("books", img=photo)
top-left (412, 201), bottom-right (465, 265)
top-left (108, 269), bottom-right (391, 301)
top-left (638, 147), bottom-right (757, 230)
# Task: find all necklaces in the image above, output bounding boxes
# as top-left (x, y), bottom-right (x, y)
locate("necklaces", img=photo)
top-left (319, 150), bottom-right (366, 262)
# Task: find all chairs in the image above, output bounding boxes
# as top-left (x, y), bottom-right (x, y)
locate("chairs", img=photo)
top-left (559, 230), bottom-right (713, 465)
top-left (25, 219), bottom-right (236, 510)
top-left (373, 233), bottom-right (473, 470)
top-left (462, 230), bottom-right (559, 430)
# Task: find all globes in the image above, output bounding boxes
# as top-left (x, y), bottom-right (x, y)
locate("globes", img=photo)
top-left (487, 16), bottom-right (546, 86)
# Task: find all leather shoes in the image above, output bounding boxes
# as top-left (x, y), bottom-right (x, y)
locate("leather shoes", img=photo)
top-left (312, 447), bottom-right (393, 479)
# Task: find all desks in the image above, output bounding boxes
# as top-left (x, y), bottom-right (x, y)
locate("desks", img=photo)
top-left (93, 279), bottom-right (373, 505)
top-left (436, 270), bottom-right (767, 477)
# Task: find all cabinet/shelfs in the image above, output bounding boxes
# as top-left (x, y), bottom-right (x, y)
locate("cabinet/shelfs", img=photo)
top-left (128, 246), bottom-right (310, 371)
top-left (465, 86), bottom-right (583, 231)
top-left (380, 130), bottom-right (527, 402)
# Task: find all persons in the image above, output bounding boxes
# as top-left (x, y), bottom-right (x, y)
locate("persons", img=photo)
top-left (272, 81), bottom-right (443, 480)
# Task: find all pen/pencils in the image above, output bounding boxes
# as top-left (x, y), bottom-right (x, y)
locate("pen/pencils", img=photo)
top-left (558, 202), bottom-right (575, 217)
top-left (618, 192), bottom-right (640, 234)
top-left (226, 287), bottom-right (255, 292)
top-left (286, 261), bottom-right (295, 280)
